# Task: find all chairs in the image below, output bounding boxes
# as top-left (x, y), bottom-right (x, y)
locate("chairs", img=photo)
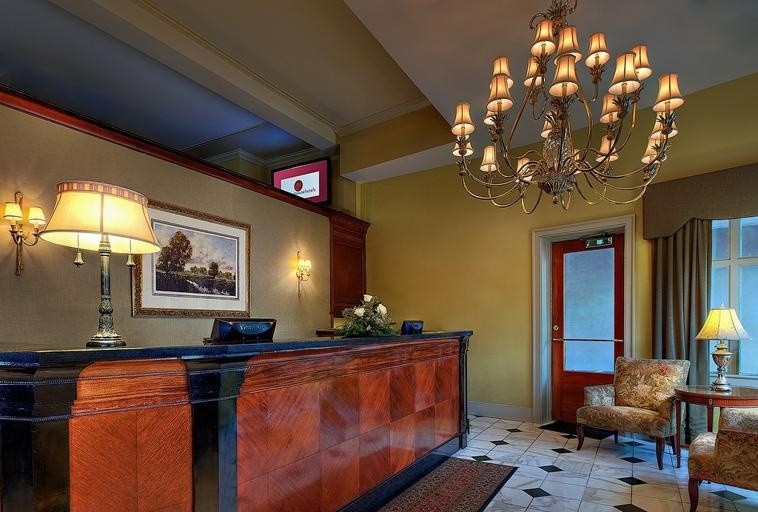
top-left (575, 356), bottom-right (691, 470)
top-left (687, 408), bottom-right (758, 512)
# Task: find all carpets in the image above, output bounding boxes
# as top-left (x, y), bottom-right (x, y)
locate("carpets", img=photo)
top-left (538, 421), bottom-right (614, 441)
top-left (376, 457), bottom-right (519, 512)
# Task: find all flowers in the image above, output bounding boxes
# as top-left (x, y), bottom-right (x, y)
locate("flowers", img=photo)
top-left (333, 294), bottom-right (398, 337)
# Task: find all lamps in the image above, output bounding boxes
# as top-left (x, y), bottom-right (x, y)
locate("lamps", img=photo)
top-left (451, 0), bottom-right (686, 214)
top-left (694, 302), bottom-right (751, 391)
top-left (39, 180), bottom-right (161, 348)
top-left (295, 248), bottom-right (312, 299)
top-left (3, 192), bottom-right (46, 276)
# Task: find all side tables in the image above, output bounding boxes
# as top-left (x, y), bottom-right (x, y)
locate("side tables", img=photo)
top-left (675, 385), bottom-right (758, 468)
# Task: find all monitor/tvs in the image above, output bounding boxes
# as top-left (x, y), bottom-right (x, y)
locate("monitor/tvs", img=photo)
top-left (400, 320), bottom-right (424, 335)
top-left (210, 318), bottom-right (277, 344)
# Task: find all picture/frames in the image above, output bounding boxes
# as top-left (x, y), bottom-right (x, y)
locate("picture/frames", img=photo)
top-left (271, 156), bottom-right (331, 204)
top-left (131, 198), bottom-right (251, 318)
